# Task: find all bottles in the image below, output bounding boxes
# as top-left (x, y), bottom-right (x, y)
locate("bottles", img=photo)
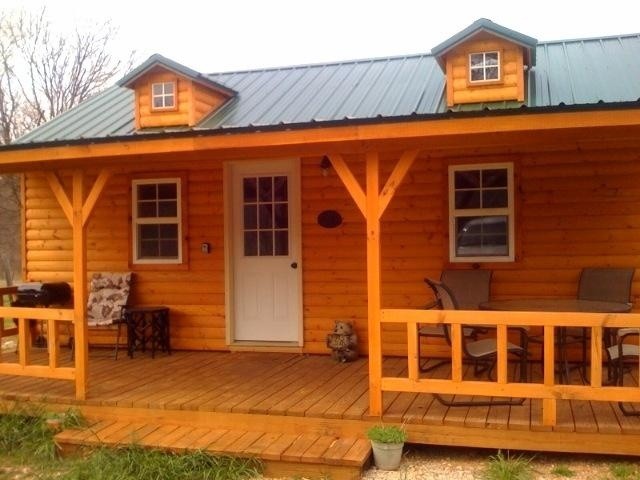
top-left (0, 340), bottom-right (18, 355)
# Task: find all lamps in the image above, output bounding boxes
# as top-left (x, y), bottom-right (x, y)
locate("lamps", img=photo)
top-left (319, 155), bottom-right (331, 177)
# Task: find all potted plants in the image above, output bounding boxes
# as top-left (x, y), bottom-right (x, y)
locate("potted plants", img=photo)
top-left (367, 425), bottom-right (406, 471)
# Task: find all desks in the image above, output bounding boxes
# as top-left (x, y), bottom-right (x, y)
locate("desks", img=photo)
top-left (125, 303), bottom-right (172, 362)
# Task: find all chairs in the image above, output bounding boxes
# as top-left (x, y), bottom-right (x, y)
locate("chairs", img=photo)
top-left (70, 269), bottom-right (135, 360)
top-left (411, 266), bottom-right (640, 417)
